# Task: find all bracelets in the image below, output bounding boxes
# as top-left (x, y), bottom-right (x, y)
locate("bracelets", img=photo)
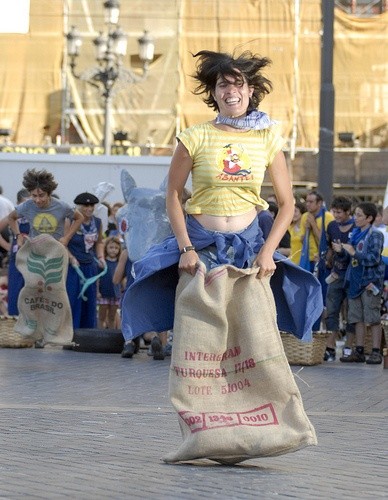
top-left (97, 256), bottom-right (104, 260)
top-left (179, 246), bottom-right (194, 254)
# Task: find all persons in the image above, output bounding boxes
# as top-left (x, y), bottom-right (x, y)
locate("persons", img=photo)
top-left (165, 49), bottom-right (296, 464)
top-left (0, 169), bottom-right (388, 369)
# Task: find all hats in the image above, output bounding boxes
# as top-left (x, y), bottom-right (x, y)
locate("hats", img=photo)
top-left (74, 192), bottom-right (99, 204)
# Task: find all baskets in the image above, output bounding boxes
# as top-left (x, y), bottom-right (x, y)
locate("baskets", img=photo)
top-left (351, 324), bottom-right (374, 354)
top-left (0, 315), bottom-right (32, 347)
top-left (279, 330), bottom-right (331, 365)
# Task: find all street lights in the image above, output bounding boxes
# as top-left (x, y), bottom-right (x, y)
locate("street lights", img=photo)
top-left (65, 0), bottom-right (155, 155)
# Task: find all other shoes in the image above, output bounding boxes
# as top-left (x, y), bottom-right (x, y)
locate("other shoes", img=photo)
top-left (343, 349), bottom-right (351, 357)
top-left (148, 347), bottom-right (153, 356)
top-left (121, 344), bottom-right (134, 358)
top-left (151, 338), bottom-right (165, 360)
top-left (325, 348), bottom-right (336, 362)
top-left (35, 339), bottom-right (43, 348)
top-left (164, 344), bottom-right (173, 354)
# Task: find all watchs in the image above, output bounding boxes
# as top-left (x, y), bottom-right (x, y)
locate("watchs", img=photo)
top-left (13, 233), bottom-right (19, 240)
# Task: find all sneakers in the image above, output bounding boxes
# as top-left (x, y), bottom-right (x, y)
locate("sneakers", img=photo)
top-left (367, 352), bottom-right (383, 364)
top-left (340, 351), bottom-right (365, 362)
